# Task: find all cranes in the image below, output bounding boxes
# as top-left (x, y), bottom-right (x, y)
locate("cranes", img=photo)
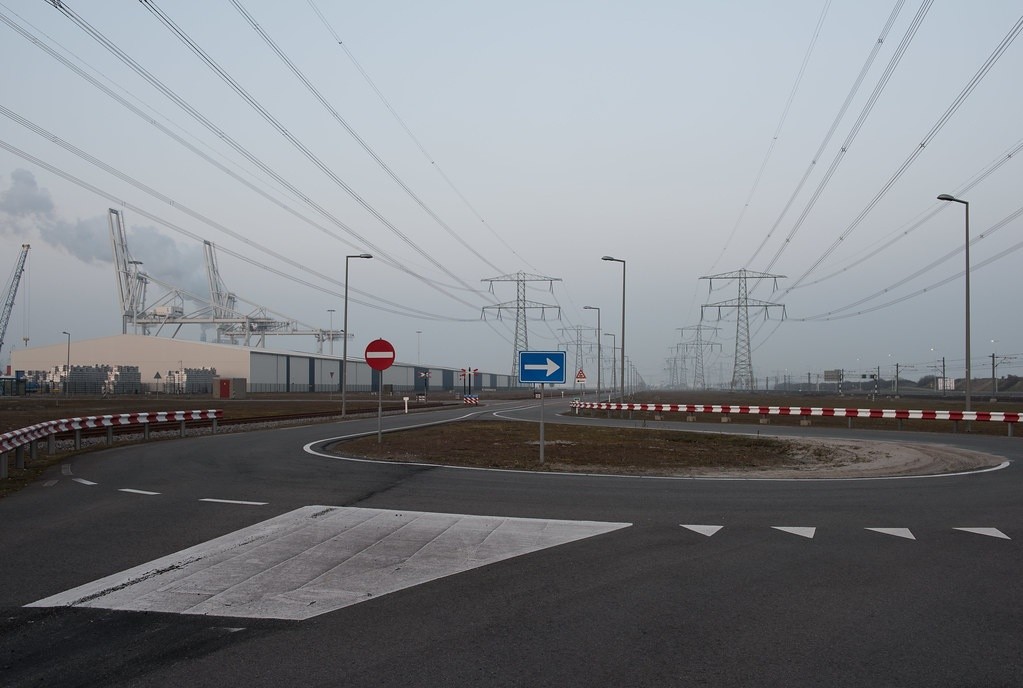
top-left (0, 242), bottom-right (32, 355)
top-left (105, 206), bottom-right (356, 355)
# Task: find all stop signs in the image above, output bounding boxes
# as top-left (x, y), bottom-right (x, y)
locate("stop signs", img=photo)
top-left (364, 339), bottom-right (396, 372)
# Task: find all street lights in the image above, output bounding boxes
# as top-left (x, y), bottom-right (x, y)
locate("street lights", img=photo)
top-left (62, 331), bottom-right (71, 396)
top-left (416, 330), bottom-right (423, 367)
top-left (624, 356), bottom-right (642, 397)
top-left (582, 306), bottom-right (602, 404)
top-left (340, 254), bottom-right (373, 419)
top-left (603, 333), bottom-right (616, 399)
top-left (326, 308), bottom-right (336, 356)
top-left (933, 192), bottom-right (972, 415)
top-left (178, 360), bottom-right (183, 395)
top-left (601, 254), bottom-right (626, 403)
